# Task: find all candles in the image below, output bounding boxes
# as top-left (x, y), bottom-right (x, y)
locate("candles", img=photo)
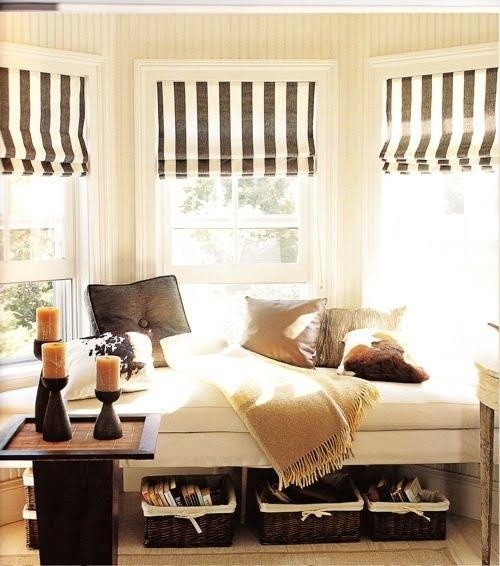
top-left (36, 306), bottom-right (122, 392)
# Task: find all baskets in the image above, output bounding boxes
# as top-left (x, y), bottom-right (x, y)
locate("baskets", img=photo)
top-left (21, 504), bottom-right (40, 550)
top-left (253, 476), bottom-right (365, 546)
top-left (139, 472), bottom-right (238, 548)
top-left (361, 488), bottom-right (451, 541)
top-left (22, 469), bottom-right (38, 511)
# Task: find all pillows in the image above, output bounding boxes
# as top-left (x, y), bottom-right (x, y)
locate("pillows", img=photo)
top-left (239, 293), bottom-right (431, 385)
top-left (82, 274), bottom-right (192, 369)
top-left (62, 332), bottom-right (154, 401)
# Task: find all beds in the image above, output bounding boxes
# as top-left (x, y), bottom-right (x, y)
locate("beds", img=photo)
top-left (1, 337), bottom-right (500, 469)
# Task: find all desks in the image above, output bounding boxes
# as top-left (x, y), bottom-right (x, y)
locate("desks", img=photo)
top-left (0, 412), bottom-right (163, 565)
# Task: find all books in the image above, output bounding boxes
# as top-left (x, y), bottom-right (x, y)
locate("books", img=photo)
top-left (141, 476), bottom-right (225, 506)
top-left (261, 478), bottom-right (336, 503)
top-left (367, 475), bottom-right (425, 502)
